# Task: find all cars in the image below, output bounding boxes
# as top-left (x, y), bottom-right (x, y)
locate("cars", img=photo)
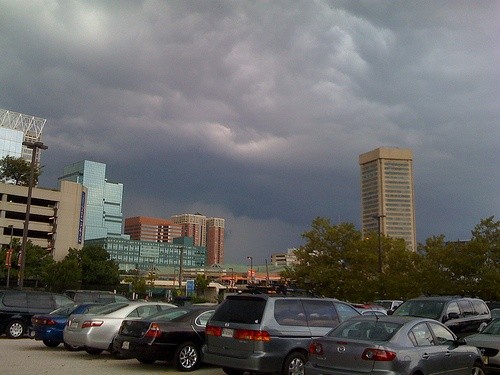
top-left (490, 308), bottom-right (500, 319)
top-left (351, 299), bottom-right (404, 316)
top-left (63, 301), bottom-right (187, 360)
top-left (305, 314), bottom-right (487, 375)
top-left (457, 318), bottom-right (500, 375)
top-left (112, 304), bottom-right (220, 372)
top-left (30, 302), bottom-right (105, 352)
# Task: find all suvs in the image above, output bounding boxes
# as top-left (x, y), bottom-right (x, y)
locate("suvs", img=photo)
top-left (203, 289), bottom-right (371, 375)
top-left (383, 294), bottom-right (492, 341)
top-left (0, 288), bottom-right (75, 339)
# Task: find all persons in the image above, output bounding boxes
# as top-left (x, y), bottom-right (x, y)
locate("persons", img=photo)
top-left (144, 295), bottom-right (149, 301)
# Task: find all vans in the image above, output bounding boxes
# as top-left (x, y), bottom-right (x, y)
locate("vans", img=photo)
top-left (73, 291), bottom-right (129, 303)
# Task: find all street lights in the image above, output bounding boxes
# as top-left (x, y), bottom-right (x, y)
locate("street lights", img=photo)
top-left (19, 141), bottom-right (49, 289)
top-left (247, 256), bottom-right (253, 283)
top-left (372, 214), bottom-right (386, 300)
top-left (6, 224), bottom-right (14, 289)
top-left (177, 247), bottom-right (184, 287)
top-left (229, 267), bottom-right (233, 288)
